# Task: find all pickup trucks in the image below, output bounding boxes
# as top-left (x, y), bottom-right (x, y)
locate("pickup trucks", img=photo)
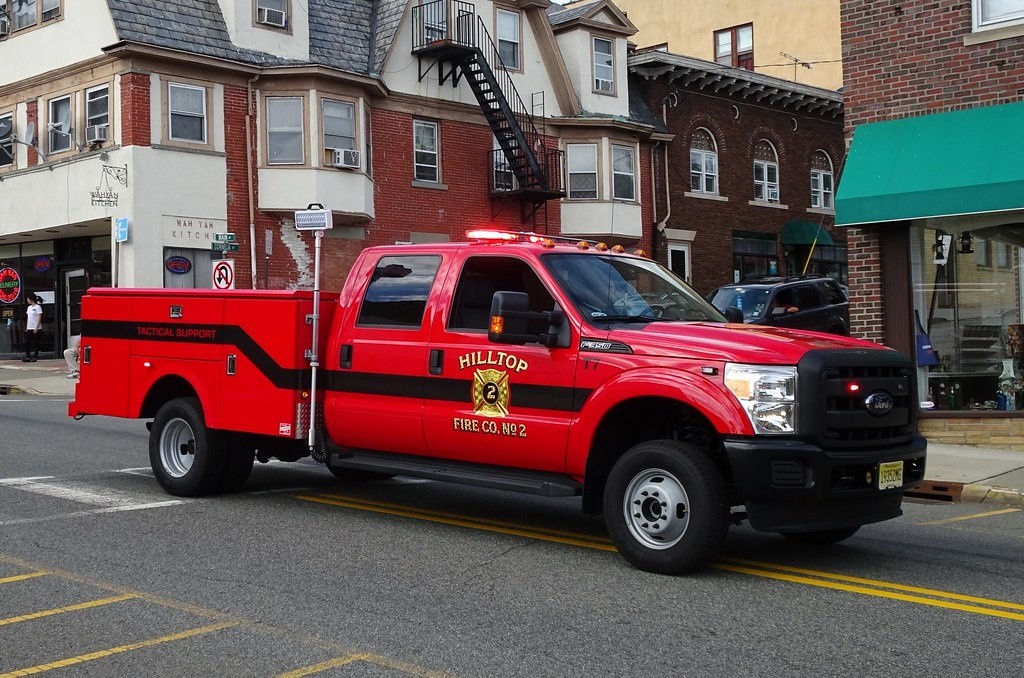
top-left (65, 231), bottom-right (928, 576)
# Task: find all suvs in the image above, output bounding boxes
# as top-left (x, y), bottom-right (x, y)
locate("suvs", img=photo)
top-left (613, 289), bottom-right (707, 324)
top-left (703, 273), bottom-right (851, 340)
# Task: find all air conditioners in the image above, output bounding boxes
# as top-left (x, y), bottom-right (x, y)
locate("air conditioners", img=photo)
top-left (260, 7), bottom-right (286, 28)
top-left (0, 19), bottom-right (8, 35)
top-left (85, 125), bottom-right (108, 142)
top-left (596, 79), bottom-right (611, 91)
top-left (767, 188), bottom-right (779, 201)
top-left (331, 149), bottom-right (360, 170)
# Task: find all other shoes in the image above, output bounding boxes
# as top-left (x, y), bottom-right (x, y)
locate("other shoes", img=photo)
top-left (30, 358), bottom-right (37, 362)
top-left (66, 371), bottom-right (80, 379)
top-left (22, 358), bottom-right (31, 362)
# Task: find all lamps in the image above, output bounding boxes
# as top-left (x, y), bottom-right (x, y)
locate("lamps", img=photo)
top-left (997, 357), bottom-right (1024, 410)
top-left (957, 231), bottom-right (974, 254)
top-left (934, 230), bottom-right (946, 260)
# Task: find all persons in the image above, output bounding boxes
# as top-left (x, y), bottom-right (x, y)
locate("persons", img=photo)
top-left (22, 293), bottom-right (44, 362)
top-left (586, 273), bottom-right (624, 316)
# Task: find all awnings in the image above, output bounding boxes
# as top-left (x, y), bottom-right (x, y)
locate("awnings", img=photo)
top-left (782, 220), bottom-right (834, 247)
top-left (834, 101), bottom-right (1024, 227)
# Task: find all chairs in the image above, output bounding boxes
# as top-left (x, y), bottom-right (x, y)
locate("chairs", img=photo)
top-left (464, 265), bottom-right (524, 328)
